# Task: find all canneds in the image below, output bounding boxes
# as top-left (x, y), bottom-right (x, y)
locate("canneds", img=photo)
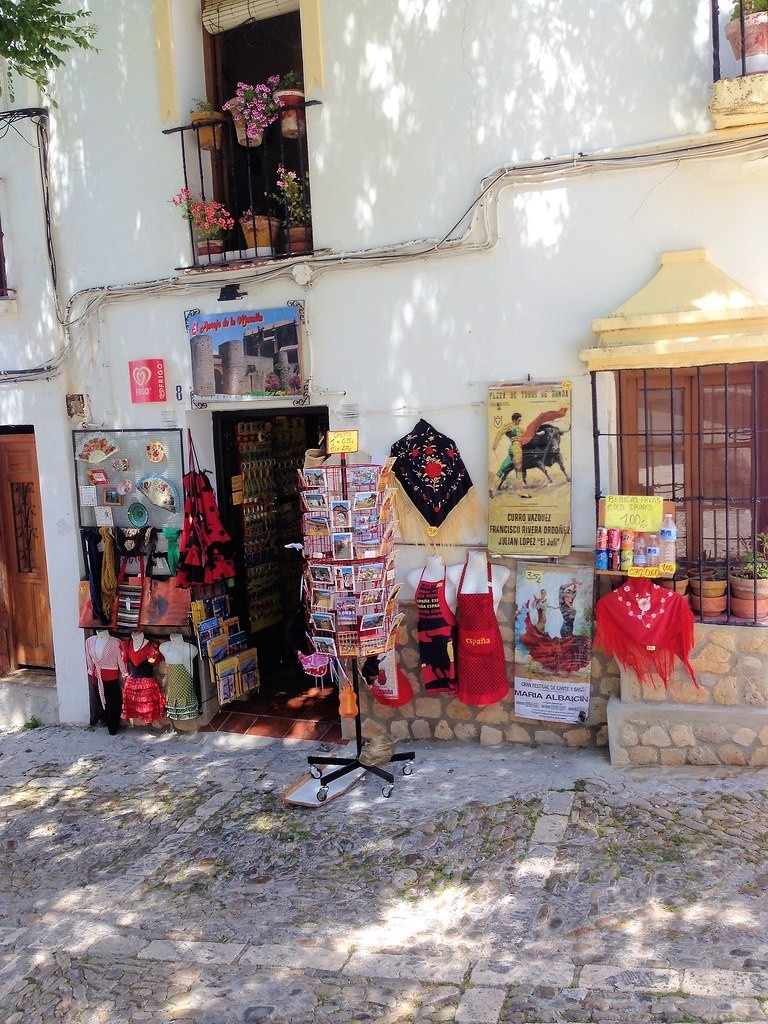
top-left (596, 527), bottom-right (634, 571)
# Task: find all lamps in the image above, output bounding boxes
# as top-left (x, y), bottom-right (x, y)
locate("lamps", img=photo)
top-left (216, 284), bottom-right (248, 301)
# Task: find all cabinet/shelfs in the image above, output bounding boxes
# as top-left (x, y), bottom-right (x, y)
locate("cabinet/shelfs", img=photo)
top-left (78, 528), bottom-right (191, 736)
top-left (297, 454), bottom-right (415, 802)
top-left (213, 408), bottom-right (328, 694)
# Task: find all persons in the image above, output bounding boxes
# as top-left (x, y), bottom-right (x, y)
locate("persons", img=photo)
top-left (118, 631), bottom-right (167, 724)
top-left (447, 550), bottom-right (511, 707)
top-left (406, 555), bottom-right (458, 696)
top-left (85, 629), bottom-right (129, 735)
top-left (159, 634), bottom-right (198, 721)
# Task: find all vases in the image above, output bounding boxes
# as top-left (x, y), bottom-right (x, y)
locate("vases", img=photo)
top-left (238, 209), bottom-right (282, 247)
top-left (284, 227), bottom-right (312, 242)
top-left (197, 239), bottom-right (223, 255)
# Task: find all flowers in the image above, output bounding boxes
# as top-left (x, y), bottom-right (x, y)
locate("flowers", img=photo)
top-left (167, 188), bottom-right (234, 239)
top-left (222, 75), bottom-right (285, 139)
top-left (263, 163), bottom-right (312, 227)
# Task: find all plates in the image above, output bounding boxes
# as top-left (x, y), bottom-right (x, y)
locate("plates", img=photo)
top-left (146, 440), bottom-right (165, 465)
top-left (127, 503), bottom-right (149, 528)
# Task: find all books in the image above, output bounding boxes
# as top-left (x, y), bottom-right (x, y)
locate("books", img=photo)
top-left (199, 616), bottom-right (260, 705)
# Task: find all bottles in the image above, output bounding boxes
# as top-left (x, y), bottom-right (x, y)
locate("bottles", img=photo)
top-left (634, 532), bottom-right (647, 568)
top-left (646, 535), bottom-right (661, 568)
top-left (660, 513), bottom-right (677, 569)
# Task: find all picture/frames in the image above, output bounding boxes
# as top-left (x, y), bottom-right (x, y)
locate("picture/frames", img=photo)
top-left (71, 427), bottom-right (186, 529)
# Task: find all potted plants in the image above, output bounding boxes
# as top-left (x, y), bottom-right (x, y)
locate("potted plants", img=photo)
top-left (686, 549), bottom-right (714, 576)
top-left (189, 96), bottom-right (223, 151)
top-left (659, 576), bottom-right (689, 596)
top-left (272, 70), bottom-right (305, 138)
top-left (729, 532), bottom-right (768, 600)
top-left (724, 0), bottom-right (768, 77)
top-left (689, 568), bottom-right (727, 597)
top-left (231, 98), bottom-right (262, 146)
top-left (676, 561), bottom-right (685, 576)
